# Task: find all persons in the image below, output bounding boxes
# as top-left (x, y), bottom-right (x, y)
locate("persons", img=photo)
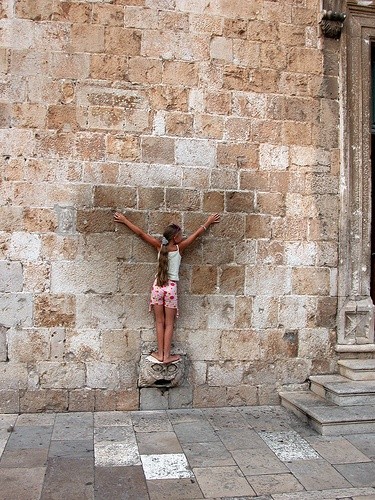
top-left (114, 211), bottom-right (221, 365)
